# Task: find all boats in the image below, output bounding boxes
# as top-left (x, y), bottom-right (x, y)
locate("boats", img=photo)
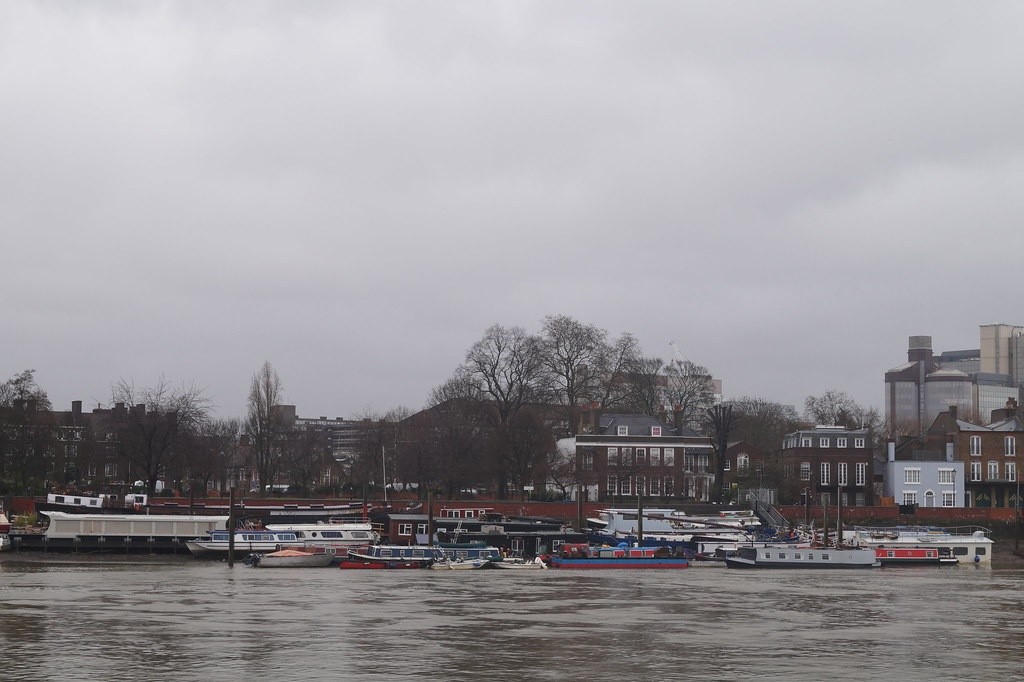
top-left (347, 545), bottom-right (501, 562)
top-left (185, 519), bottom-right (380, 556)
top-left (876, 548), bottom-right (959, 565)
top-left (243, 547), bottom-right (336, 567)
top-left (539, 544), bottom-right (688, 569)
top-left (430, 559), bottom-right (489, 570)
top-left (340, 559), bottom-right (419, 569)
top-left (491, 556), bottom-right (548, 570)
top-left (724, 547), bottom-right (876, 569)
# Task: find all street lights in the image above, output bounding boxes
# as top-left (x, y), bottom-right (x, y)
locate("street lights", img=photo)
top-left (951, 469), bottom-right (957, 506)
